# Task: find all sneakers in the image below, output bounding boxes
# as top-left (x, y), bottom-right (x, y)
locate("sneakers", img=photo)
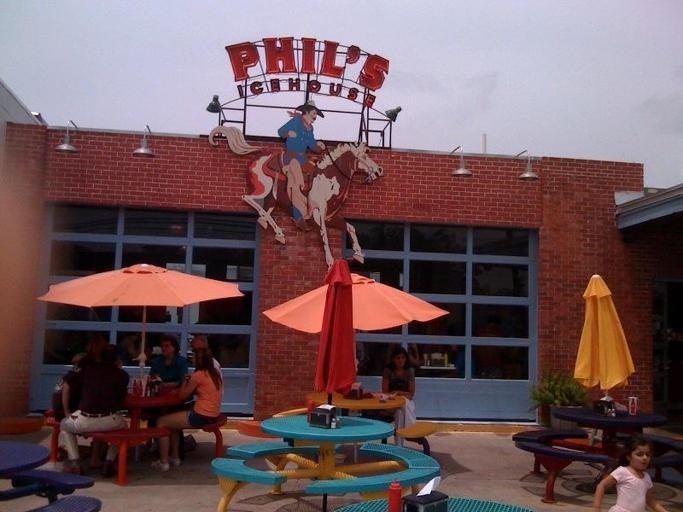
top-left (62, 462), bottom-right (82, 474)
top-left (165, 455), bottom-right (181, 466)
top-left (100, 460), bottom-right (115, 476)
top-left (150, 459), bottom-right (170, 472)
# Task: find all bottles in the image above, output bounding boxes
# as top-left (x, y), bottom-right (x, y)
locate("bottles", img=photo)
top-left (330, 418), bottom-right (337, 429)
top-left (306, 399), bottom-right (314, 424)
top-left (422, 353), bottom-right (430, 366)
top-left (127, 375), bottom-right (160, 397)
top-left (443, 352), bottom-right (448, 366)
top-left (630, 398), bottom-right (635, 415)
top-left (387, 478), bottom-right (401, 511)
top-left (607, 408), bottom-right (616, 417)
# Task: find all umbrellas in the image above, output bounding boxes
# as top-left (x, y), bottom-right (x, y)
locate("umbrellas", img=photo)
top-left (34, 263), bottom-right (245, 377)
top-left (313, 260), bottom-right (357, 403)
top-left (263, 273), bottom-right (451, 334)
top-left (573, 274), bottom-right (636, 394)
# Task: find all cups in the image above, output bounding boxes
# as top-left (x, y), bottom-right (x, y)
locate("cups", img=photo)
top-left (627, 396), bottom-right (638, 415)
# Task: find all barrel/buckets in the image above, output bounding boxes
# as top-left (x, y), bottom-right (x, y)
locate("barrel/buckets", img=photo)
top-left (548, 404), bottom-right (583, 431)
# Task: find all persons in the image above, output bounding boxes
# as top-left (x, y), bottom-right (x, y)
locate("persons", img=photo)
top-left (278, 101), bottom-right (325, 223)
top-left (594, 433), bottom-right (666, 512)
top-left (382, 349), bottom-right (415, 447)
top-left (54, 331), bottom-right (222, 472)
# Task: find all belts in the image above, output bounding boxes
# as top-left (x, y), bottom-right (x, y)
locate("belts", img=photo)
top-left (80, 411), bottom-right (117, 418)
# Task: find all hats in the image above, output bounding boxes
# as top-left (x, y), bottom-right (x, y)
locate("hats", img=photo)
top-left (295, 100), bottom-right (325, 118)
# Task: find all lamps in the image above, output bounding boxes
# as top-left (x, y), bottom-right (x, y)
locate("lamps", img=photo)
top-left (206, 96), bottom-right (226, 126)
top-left (133, 124), bottom-right (155, 159)
top-left (380, 106), bottom-right (402, 135)
top-left (53, 119), bottom-right (78, 153)
top-left (448, 145), bottom-right (473, 176)
top-left (515, 149), bottom-right (540, 181)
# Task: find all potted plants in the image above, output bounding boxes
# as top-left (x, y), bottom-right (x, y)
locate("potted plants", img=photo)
top-left (527, 368), bottom-right (589, 431)
